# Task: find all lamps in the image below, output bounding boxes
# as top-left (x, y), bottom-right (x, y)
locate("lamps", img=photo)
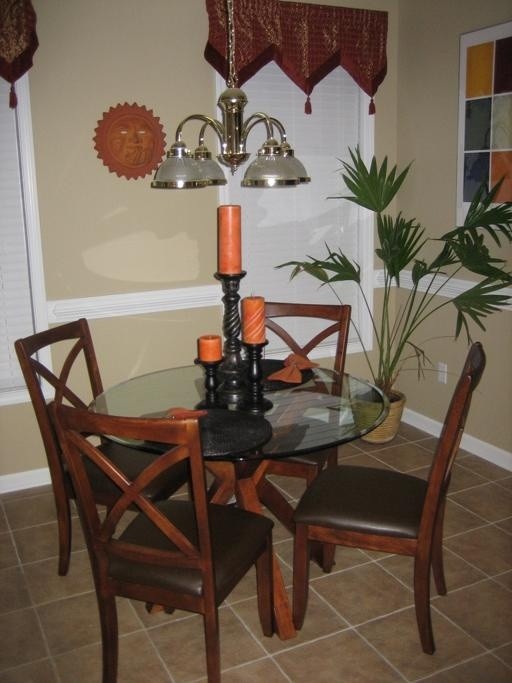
top-left (150, 0), bottom-right (310, 190)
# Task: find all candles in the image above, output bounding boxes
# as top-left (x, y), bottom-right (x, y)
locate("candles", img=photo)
top-left (240, 296), bottom-right (266, 344)
top-left (216, 204), bottom-right (243, 275)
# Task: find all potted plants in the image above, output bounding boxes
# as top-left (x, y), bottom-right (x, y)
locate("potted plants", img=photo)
top-left (270, 142), bottom-right (512, 445)
